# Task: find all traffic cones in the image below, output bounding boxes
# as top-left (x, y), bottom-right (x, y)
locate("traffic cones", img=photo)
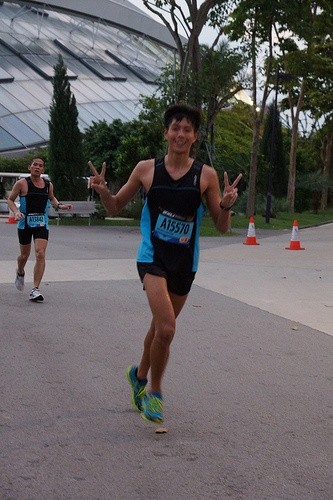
top-left (5, 209), bottom-right (17, 224)
top-left (285, 219), bottom-right (305, 250)
top-left (241, 216), bottom-right (260, 245)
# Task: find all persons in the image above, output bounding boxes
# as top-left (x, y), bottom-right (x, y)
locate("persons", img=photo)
top-left (83, 104), bottom-right (244, 426)
top-left (6, 155), bottom-right (72, 302)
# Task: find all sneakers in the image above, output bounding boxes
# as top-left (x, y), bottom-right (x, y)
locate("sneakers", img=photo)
top-left (139, 391), bottom-right (163, 425)
top-left (14, 272), bottom-right (25, 290)
top-left (127, 366), bottom-right (148, 413)
top-left (29, 287), bottom-right (44, 302)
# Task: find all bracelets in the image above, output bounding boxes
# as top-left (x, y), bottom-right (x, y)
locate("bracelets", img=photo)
top-left (219, 201), bottom-right (233, 212)
top-left (57, 203), bottom-right (62, 209)
